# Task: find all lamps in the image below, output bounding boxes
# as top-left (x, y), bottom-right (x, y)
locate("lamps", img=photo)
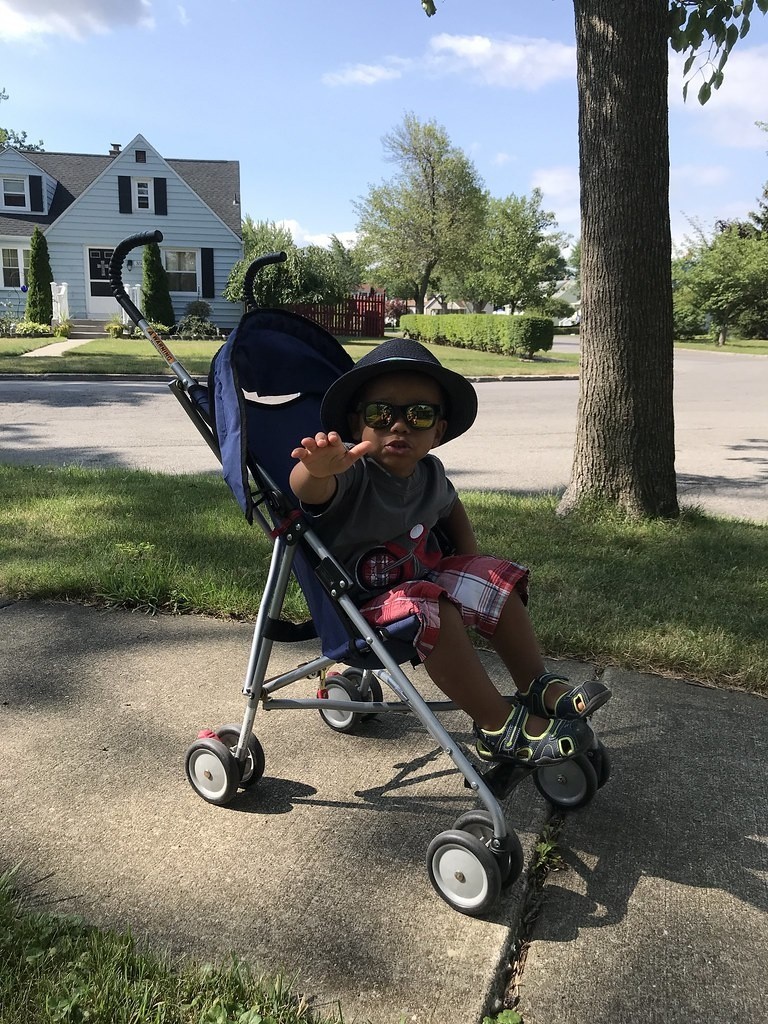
top-left (126, 260), bottom-right (133, 271)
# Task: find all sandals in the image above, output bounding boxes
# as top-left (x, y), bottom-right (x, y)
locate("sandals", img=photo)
top-left (514, 674), bottom-right (612, 719)
top-left (476, 703), bottom-right (594, 767)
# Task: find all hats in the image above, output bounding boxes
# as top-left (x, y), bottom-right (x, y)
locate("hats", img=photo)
top-left (320, 338), bottom-right (477, 449)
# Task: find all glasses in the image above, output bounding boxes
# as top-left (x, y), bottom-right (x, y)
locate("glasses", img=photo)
top-left (357, 400), bottom-right (443, 431)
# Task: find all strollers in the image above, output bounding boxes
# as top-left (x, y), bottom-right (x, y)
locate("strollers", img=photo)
top-left (108, 230), bottom-right (614, 920)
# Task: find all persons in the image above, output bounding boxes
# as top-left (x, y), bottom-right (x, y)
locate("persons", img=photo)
top-left (289, 338), bottom-right (613, 768)
